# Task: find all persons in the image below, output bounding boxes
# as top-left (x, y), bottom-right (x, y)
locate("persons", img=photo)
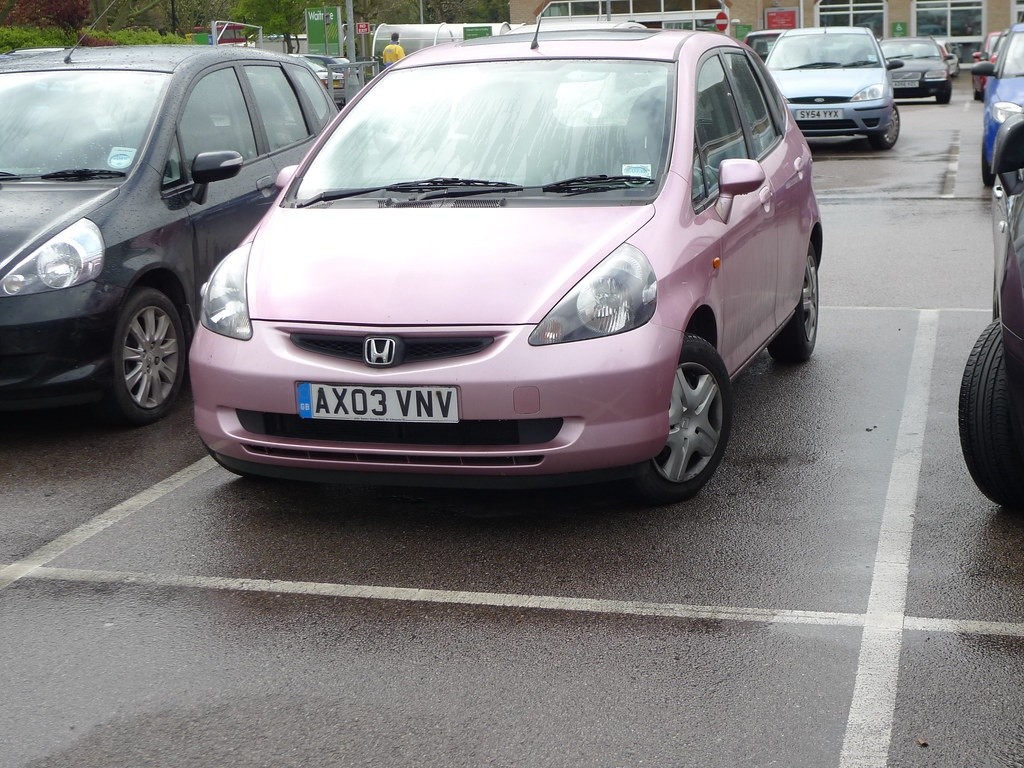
top-left (382, 33), bottom-right (406, 68)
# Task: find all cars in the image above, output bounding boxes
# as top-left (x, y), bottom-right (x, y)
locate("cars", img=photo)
top-left (969, 21), bottom-right (1024, 187)
top-left (761, 25), bottom-right (905, 151)
top-left (957, 110), bottom-right (1023, 511)
top-left (744, 28), bottom-right (787, 66)
top-left (874, 34), bottom-right (961, 105)
top-left (187, 32), bottom-right (825, 507)
top-left (0, 29), bottom-right (360, 428)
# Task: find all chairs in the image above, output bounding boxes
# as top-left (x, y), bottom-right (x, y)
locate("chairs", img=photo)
top-left (621, 85), bottom-right (710, 173)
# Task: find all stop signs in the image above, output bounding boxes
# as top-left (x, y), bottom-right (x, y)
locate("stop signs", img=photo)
top-left (714, 12), bottom-right (729, 32)
